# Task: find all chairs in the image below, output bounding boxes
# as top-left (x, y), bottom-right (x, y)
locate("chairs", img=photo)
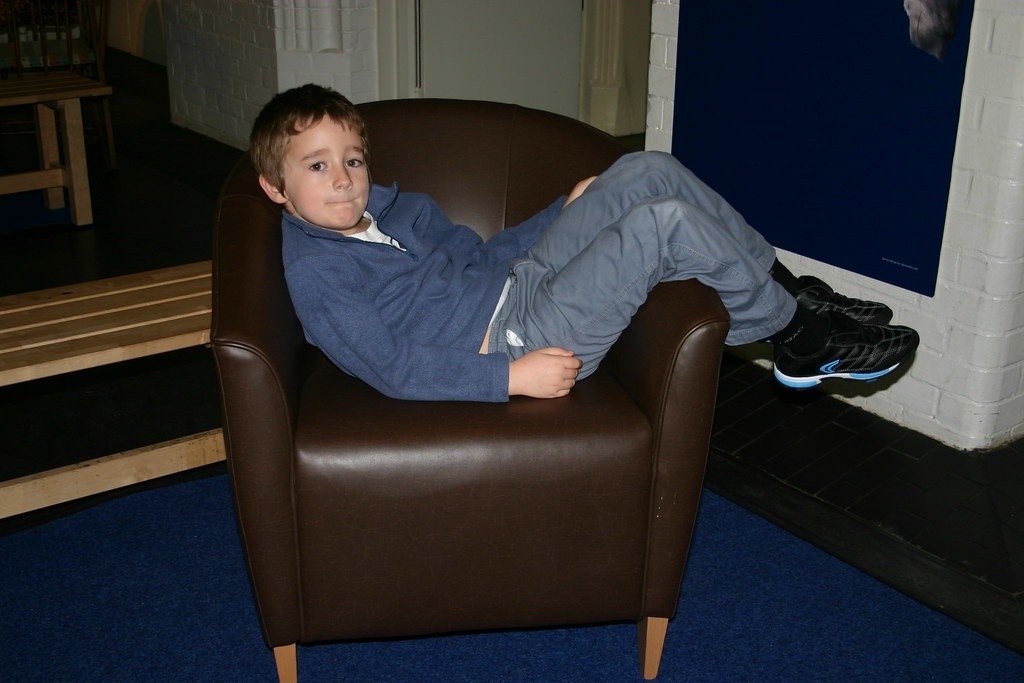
top-left (0, 0), bottom-right (120, 170)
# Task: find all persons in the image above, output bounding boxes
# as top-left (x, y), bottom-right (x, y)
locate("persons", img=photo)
top-left (251, 84), bottom-right (920, 402)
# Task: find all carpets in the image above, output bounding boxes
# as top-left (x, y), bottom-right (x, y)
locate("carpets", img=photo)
top-left (0, 471), bottom-right (1024, 683)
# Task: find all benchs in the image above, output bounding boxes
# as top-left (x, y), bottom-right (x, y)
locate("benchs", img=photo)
top-left (0, 69), bottom-right (114, 227)
top-left (0, 258), bottom-right (229, 525)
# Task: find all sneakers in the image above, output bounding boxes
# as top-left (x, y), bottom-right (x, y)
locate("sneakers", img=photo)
top-left (767, 275), bottom-right (894, 342)
top-left (772, 310), bottom-right (920, 388)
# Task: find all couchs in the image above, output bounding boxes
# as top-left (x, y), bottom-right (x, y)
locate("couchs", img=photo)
top-left (211, 97), bottom-right (732, 683)
